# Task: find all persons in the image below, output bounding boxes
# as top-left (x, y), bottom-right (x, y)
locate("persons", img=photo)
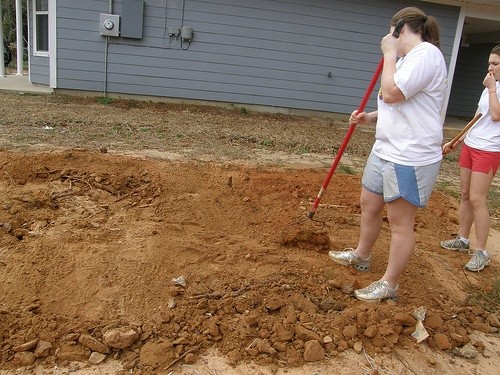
top-left (440, 44), bottom-right (500, 272)
top-left (327, 6), bottom-right (448, 303)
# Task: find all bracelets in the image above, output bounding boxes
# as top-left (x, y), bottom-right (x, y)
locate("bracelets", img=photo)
top-left (489, 91), bottom-right (497, 95)
top-left (457, 139), bottom-right (461, 143)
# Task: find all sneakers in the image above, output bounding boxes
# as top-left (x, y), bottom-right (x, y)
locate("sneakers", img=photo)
top-left (441, 235), bottom-right (470, 251)
top-left (328, 248), bottom-right (371, 272)
top-left (465, 249), bottom-right (491, 271)
top-left (354, 280), bottom-right (399, 302)
